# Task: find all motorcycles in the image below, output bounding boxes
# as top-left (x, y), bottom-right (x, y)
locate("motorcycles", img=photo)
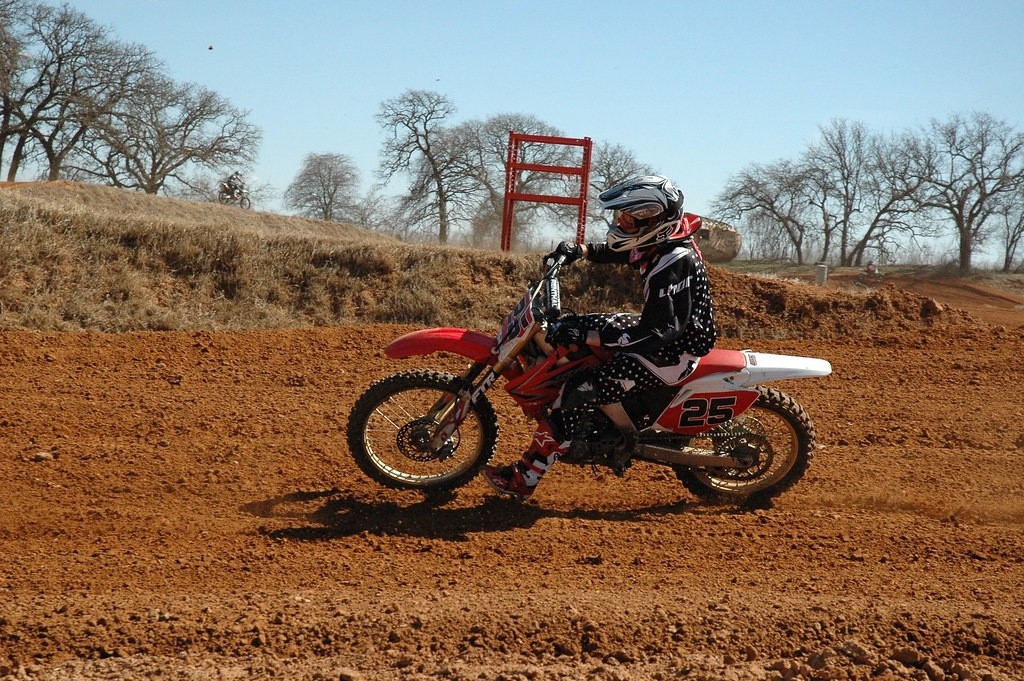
top-left (219, 181), bottom-right (250, 209)
top-left (345, 253), bottom-right (831, 502)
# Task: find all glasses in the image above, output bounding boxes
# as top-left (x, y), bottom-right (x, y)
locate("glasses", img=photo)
top-left (617, 208), bottom-right (643, 234)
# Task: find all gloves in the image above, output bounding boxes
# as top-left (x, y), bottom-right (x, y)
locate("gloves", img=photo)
top-left (555, 241), bottom-right (583, 267)
top-left (544, 314), bottom-right (585, 350)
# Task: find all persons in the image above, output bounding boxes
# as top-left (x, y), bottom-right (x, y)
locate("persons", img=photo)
top-left (482, 175), bottom-right (717, 501)
top-left (866, 260), bottom-right (877, 278)
top-left (228, 170), bottom-right (246, 198)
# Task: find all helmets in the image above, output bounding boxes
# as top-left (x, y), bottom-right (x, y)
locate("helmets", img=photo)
top-left (597, 175), bottom-right (683, 255)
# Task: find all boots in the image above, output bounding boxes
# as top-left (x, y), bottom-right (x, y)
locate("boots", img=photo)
top-left (480, 419), bottom-right (574, 504)
